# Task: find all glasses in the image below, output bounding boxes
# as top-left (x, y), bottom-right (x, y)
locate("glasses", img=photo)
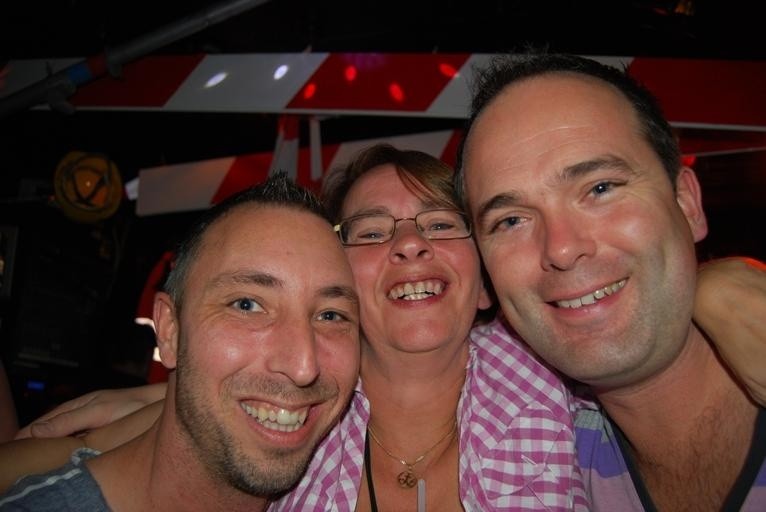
top-left (333, 209), bottom-right (473, 246)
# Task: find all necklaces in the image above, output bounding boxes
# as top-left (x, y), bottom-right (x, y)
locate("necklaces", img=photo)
top-left (368, 426), bottom-right (455, 490)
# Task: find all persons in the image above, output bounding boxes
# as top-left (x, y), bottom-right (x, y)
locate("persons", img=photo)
top-left (1, 180), bottom-right (361, 511)
top-left (12, 142), bottom-right (766, 511)
top-left (454, 49), bottom-right (764, 511)
